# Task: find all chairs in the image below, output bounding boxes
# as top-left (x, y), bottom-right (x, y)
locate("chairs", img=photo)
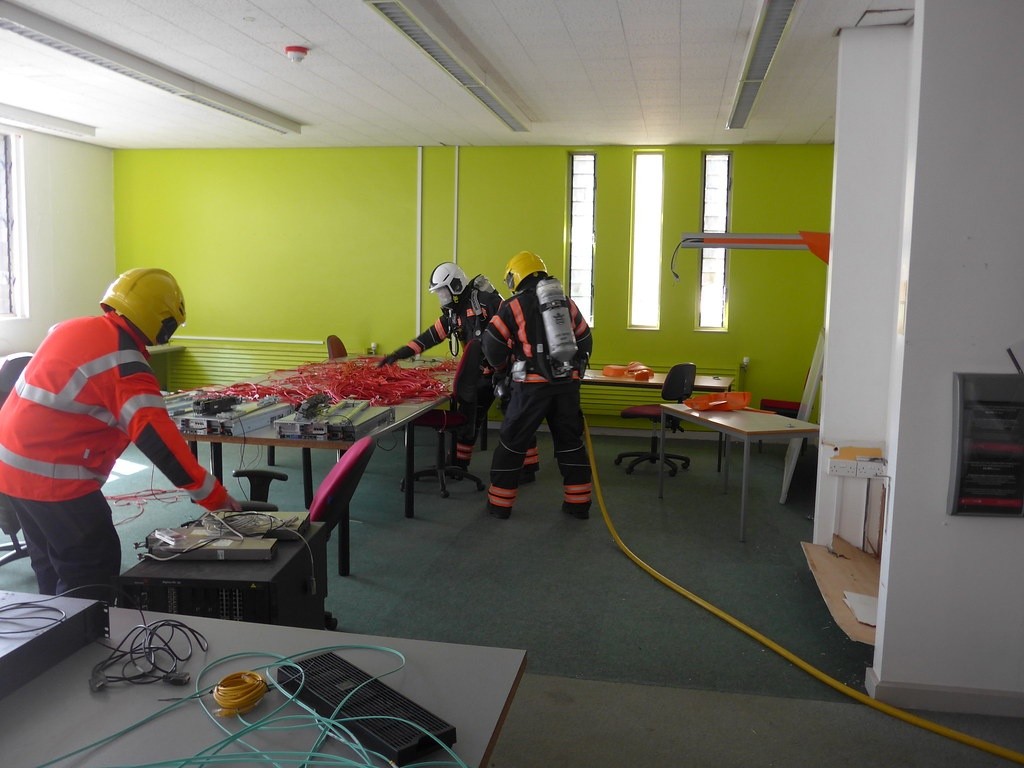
top-left (615, 361), bottom-right (695, 476)
top-left (0, 353), bottom-right (35, 569)
top-left (326, 335), bottom-right (348, 361)
top-left (400, 337), bottom-right (507, 500)
top-left (181, 434), bottom-right (376, 599)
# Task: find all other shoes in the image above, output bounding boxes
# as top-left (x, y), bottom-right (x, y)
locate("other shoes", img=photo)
top-left (518, 471), bottom-right (536, 486)
top-left (573, 512), bottom-right (589, 519)
top-left (458, 464), bottom-right (468, 473)
top-left (488, 510), bottom-right (511, 518)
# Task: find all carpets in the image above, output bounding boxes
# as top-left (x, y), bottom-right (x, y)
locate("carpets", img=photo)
top-left (0, 428), bottom-right (874, 703)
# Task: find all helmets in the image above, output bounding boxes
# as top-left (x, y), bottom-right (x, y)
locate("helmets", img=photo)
top-left (100, 267), bottom-right (186, 346)
top-left (502, 250), bottom-right (548, 296)
top-left (428, 262), bottom-right (469, 296)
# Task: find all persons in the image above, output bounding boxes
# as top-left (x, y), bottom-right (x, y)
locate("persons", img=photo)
top-left (374, 262), bottom-right (540, 488)
top-left (1, 266), bottom-right (241, 605)
top-left (481, 251), bottom-right (594, 519)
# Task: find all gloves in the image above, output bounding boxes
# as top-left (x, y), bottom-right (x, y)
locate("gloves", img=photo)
top-left (376, 354), bottom-right (398, 368)
top-left (492, 368), bottom-right (509, 389)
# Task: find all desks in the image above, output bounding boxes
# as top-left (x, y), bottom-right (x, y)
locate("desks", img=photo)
top-left (0, 603), bottom-right (526, 768)
top-left (579, 368), bottom-right (737, 473)
top-left (658, 403), bottom-right (820, 544)
top-left (181, 354), bottom-right (487, 575)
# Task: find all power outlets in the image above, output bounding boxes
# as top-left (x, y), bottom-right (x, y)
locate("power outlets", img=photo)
top-left (857, 462), bottom-right (885, 477)
top-left (827, 459), bottom-right (857, 477)
